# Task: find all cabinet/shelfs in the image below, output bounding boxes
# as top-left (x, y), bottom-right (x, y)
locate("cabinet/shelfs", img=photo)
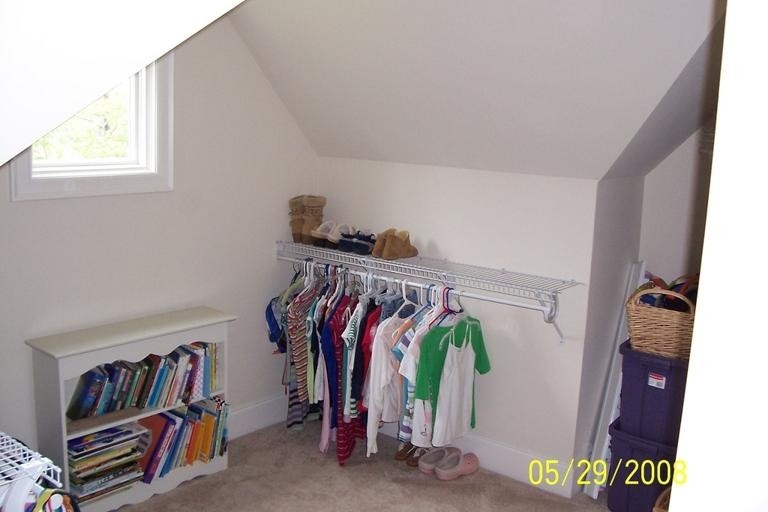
top-left (23, 308), bottom-right (239, 511)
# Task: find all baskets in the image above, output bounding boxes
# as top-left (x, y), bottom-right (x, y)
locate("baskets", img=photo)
top-left (625, 288), bottom-right (695, 360)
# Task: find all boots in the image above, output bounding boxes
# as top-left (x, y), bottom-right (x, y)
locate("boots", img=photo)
top-left (289, 195), bottom-right (325, 244)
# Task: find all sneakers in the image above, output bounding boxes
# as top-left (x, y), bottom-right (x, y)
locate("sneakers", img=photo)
top-left (315, 220), bottom-right (418, 261)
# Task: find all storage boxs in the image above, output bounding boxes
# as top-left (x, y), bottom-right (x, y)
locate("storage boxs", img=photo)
top-left (606, 340), bottom-right (688, 512)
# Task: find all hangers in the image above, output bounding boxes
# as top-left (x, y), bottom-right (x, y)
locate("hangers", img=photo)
top-left (277, 260), bottom-right (480, 349)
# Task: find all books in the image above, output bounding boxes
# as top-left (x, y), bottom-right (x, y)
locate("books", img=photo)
top-left (68, 395), bottom-right (231, 505)
top-left (65, 342), bottom-right (224, 420)
